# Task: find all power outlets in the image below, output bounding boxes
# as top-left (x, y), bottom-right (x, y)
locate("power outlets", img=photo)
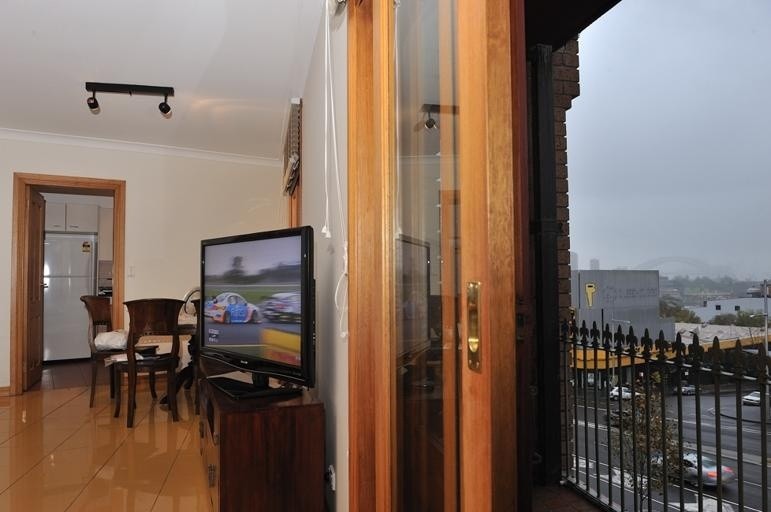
top-left (327, 465), bottom-right (336, 491)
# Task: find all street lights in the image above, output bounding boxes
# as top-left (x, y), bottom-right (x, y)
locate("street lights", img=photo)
top-left (611, 319), bottom-right (632, 383)
top-left (747, 312), bottom-right (771, 397)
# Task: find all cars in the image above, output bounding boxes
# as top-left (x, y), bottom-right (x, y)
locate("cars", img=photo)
top-left (649, 450), bottom-right (738, 491)
top-left (608, 385), bottom-right (642, 402)
top-left (672, 383), bottom-right (703, 397)
top-left (742, 390), bottom-right (761, 406)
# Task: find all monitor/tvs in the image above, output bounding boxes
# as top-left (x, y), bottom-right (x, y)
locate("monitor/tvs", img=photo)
top-left (197, 226), bottom-right (317, 400)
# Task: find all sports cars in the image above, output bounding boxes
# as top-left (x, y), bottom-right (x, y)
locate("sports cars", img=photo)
top-left (204, 290), bottom-right (261, 327)
top-left (258, 291), bottom-right (301, 324)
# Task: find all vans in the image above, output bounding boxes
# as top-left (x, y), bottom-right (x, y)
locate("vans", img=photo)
top-left (588, 372), bottom-right (595, 386)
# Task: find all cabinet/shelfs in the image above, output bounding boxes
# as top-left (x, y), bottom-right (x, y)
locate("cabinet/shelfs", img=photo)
top-left (192, 350), bottom-right (330, 512)
top-left (43, 199), bottom-right (102, 235)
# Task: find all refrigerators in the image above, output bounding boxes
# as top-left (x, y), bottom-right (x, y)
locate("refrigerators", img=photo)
top-left (44, 230), bottom-right (97, 362)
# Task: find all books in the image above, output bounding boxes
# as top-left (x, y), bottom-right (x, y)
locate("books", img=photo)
top-left (104, 352), bottom-right (144, 368)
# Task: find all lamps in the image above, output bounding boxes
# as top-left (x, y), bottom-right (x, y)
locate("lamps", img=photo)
top-left (420, 103), bottom-right (458, 131)
top-left (82, 81), bottom-right (179, 119)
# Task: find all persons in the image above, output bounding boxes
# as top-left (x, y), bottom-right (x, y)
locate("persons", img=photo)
top-left (230, 296), bottom-right (237, 305)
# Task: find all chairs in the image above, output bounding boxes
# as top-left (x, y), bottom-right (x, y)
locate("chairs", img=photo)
top-left (112, 294), bottom-right (183, 429)
top-left (77, 291), bottom-right (158, 410)
